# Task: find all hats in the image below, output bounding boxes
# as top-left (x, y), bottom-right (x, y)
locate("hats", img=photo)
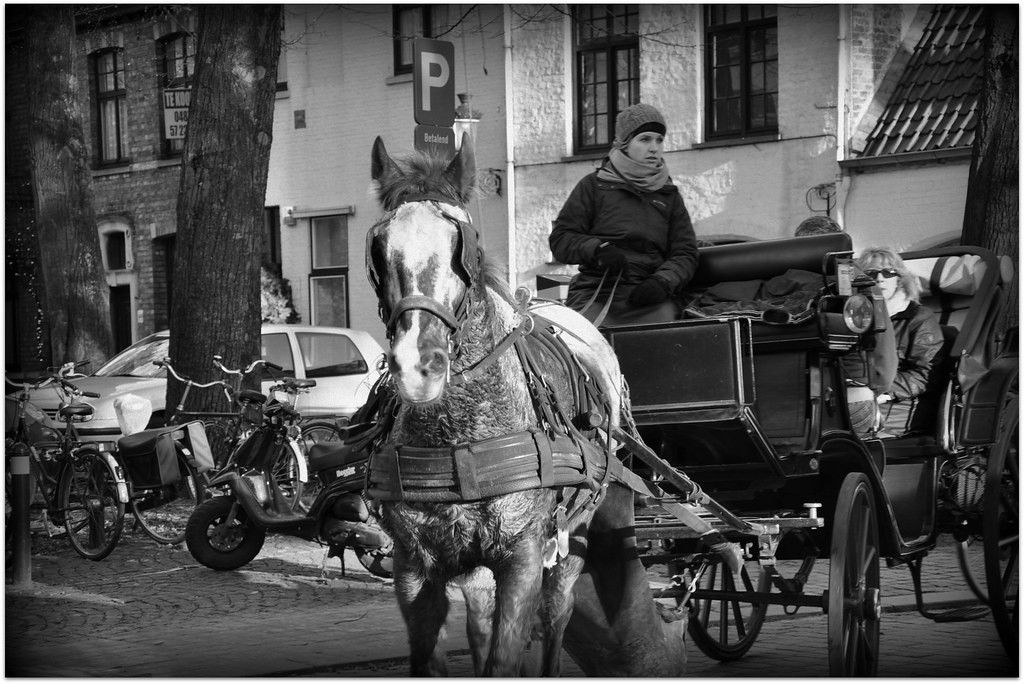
top-left (616, 103), bottom-right (666, 144)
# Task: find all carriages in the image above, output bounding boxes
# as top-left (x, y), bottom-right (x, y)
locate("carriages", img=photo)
top-left (339, 128), bottom-right (1019, 677)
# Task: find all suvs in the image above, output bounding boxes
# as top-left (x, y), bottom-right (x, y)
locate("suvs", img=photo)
top-left (5, 323), bottom-right (392, 456)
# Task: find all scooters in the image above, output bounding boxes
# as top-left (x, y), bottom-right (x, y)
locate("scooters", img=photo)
top-left (183, 401), bottom-right (396, 579)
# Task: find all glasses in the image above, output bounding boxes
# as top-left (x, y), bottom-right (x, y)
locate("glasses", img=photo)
top-left (864, 268), bottom-right (901, 279)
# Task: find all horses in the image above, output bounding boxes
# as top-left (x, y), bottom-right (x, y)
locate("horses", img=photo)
top-left (367, 131), bottom-right (620, 678)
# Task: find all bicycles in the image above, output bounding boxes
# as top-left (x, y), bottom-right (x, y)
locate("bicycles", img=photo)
top-left (6, 354), bottom-right (351, 560)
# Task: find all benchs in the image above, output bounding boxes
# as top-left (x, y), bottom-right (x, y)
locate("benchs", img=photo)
top-left (677, 232), bottom-right (877, 354)
top-left (880, 325), bottom-right (960, 465)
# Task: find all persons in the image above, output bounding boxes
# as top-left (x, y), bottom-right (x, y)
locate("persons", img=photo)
top-left (547, 104), bottom-right (699, 328)
top-left (795, 214), bottom-right (945, 439)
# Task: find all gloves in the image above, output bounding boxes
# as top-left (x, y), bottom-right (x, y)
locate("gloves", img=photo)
top-left (594, 241), bottom-right (629, 273)
top-left (626, 274), bottom-right (670, 311)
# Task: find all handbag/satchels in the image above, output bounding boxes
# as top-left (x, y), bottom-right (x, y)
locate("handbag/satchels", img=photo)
top-left (118, 419), bottom-right (215, 490)
